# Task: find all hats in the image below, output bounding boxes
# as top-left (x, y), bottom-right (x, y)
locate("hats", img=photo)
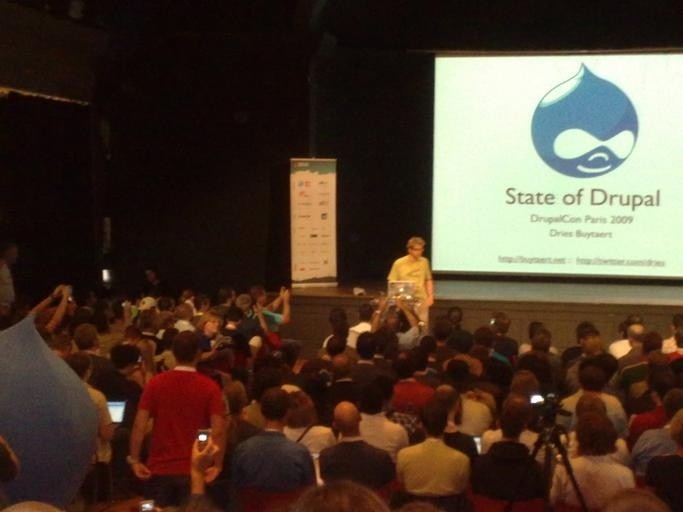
top-left (138, 296), bottom-right (157, 311)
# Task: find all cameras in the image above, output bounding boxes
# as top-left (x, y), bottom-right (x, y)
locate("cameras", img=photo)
top-left (531, 394), bottom-right (572, 425)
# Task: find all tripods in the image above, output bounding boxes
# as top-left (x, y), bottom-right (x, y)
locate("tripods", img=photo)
top-left (507, 425), bottom-right (586, 512)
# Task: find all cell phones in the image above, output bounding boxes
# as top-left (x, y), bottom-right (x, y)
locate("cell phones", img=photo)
top-left (140, 500), bottom-right (154, 511)
top-left (198, 429), bottom-right (210, 450)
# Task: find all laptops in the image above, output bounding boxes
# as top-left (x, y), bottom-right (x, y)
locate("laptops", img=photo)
top-left (105, 399), bottom-right (126, 428)
top-left (389, 280), bottom-right (417, 299)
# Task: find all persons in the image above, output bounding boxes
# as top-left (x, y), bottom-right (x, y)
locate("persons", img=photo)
top-left (0, 242), bottom-right (682, 512)
top-left (386, 237), bottom-right (433, 333)
